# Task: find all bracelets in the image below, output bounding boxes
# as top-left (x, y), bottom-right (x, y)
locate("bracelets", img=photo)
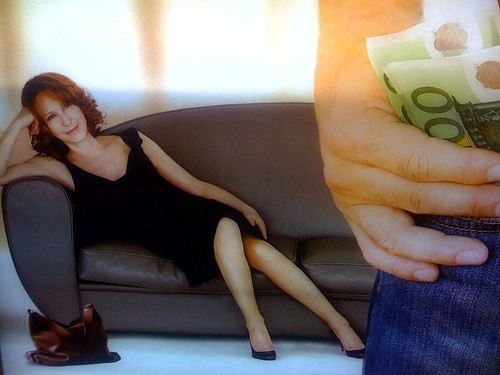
top-left (2, 132), bottom-right (17, 144)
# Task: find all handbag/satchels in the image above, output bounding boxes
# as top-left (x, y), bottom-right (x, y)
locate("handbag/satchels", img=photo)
top-left (25, 304), bottom-right (120, 367)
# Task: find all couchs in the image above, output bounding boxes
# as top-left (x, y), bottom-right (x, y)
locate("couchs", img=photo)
top-left (2, 103), bottom-right (380, 340)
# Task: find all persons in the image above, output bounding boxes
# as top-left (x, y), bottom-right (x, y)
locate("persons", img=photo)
top-left (314, 0), bottom-right (500, 375)
top-left (0, 72), bottom-right (366, 360)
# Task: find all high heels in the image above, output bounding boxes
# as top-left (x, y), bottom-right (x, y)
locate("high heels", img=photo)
top-left (248, 334), bottom-right (276, 360)
top-left (340, 340), bottom-right (367, 359)
